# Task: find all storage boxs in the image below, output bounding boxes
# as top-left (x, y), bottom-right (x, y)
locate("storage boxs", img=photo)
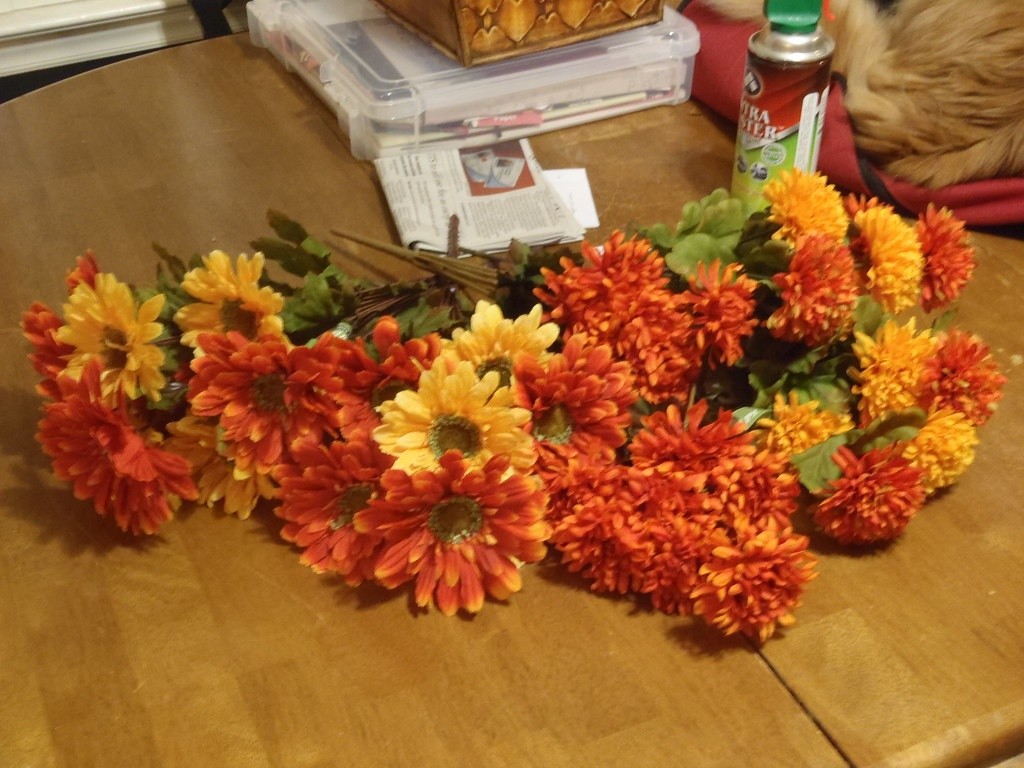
top-left (373, 0), bottom-right (663, 69)
top-left (246, 0), bottom-right (702, 163)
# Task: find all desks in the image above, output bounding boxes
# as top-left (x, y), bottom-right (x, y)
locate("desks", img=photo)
top-left (529, 103), bottom-right (1024, 768)
top-left (0, 31), bottom-right (848, 768)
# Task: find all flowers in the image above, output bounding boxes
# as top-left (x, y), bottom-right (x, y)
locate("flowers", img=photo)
top-left (17, 167), bottom-right (1011, 644)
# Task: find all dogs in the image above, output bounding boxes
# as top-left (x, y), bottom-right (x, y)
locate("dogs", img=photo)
top-left (699, 0), bottom-right (1024, 193)
